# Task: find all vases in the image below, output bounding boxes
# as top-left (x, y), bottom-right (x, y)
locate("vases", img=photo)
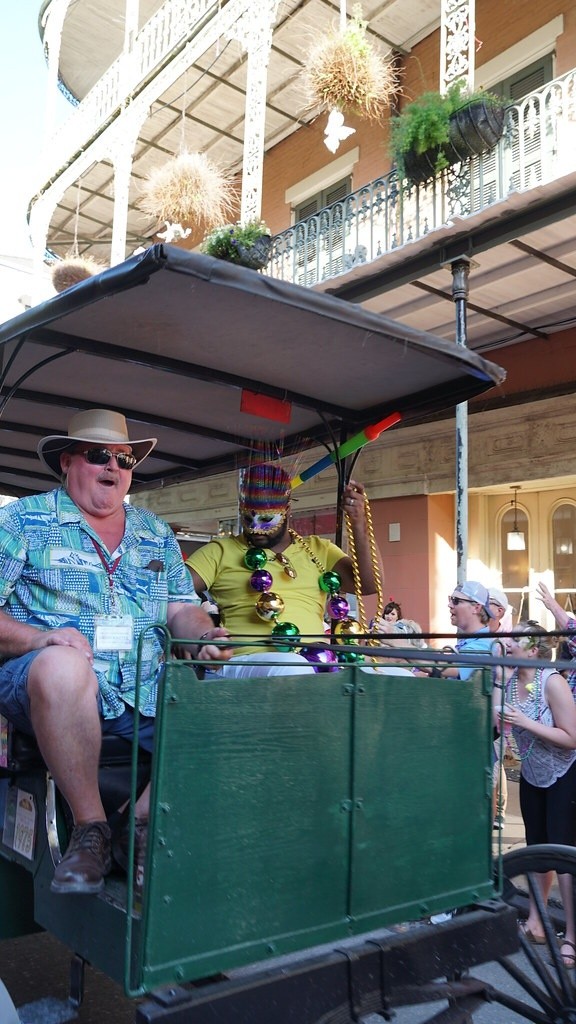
top-left (220, 235), bottom-right (270, 270)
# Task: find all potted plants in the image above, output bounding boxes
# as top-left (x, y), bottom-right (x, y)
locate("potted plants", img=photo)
top-left (376, 79), bottom-right (508, 189)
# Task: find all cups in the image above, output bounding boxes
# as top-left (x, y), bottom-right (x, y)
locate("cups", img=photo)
top-left (495, 706), bottom-right (512, 736)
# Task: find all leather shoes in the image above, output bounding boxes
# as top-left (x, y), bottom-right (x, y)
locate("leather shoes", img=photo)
top-left (119, 816), bottom-right (148, 905)
top-left (49, 820), bottom-right (112, 894)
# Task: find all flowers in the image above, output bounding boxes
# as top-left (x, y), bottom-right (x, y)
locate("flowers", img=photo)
top-left (525, 683), bottom-right (534, 692)
top-left (518, 626), bottom-right (540, 651)
top-left (202, 218), bottom-right (272, 254)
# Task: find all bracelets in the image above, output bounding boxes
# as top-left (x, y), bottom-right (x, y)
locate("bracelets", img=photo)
top-left (196, 633), bottom-right (208, 654)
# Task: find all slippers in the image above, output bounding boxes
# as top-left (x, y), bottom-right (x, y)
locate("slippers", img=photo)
top-left (520, 923), bottom-right (547, 945)
top-left (551, 941), bottom-right (576, 969)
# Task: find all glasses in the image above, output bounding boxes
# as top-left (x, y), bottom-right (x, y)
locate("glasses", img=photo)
top-left (68, 447), bottom-right (136, 469)
top-left (275, 553), bottom-right (298, 579)
top-left (394, 623), bottom-right (410, 645)
top-left (527, 620), bottom-right (540, 649)
top-left (448, 596), bottom-right (478, 605)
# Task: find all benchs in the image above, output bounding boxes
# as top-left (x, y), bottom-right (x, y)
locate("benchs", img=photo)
top-left (8, 733), bottom-right (151, 863)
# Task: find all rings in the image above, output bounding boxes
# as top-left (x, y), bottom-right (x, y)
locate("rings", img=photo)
top-left (350, 499), bottom-right (354, 505)
top-left (509, 717), bottom-right (512, 720)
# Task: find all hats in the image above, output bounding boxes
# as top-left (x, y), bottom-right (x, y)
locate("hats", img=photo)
top-left (488, 587), bottom-right (508, 611)
top-left (37, 409), bottom-right (157, 481)
top-left (454, 580), bottom-right (496, 619)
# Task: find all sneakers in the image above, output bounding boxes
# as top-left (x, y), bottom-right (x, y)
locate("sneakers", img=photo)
top-left (493, 815), bottom-right (505, 829)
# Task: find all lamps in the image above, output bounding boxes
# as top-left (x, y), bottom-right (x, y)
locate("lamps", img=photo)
top-left (555, 510), bottom-right (573, 555)
top-left (506, 485), bottom-right (525, 551)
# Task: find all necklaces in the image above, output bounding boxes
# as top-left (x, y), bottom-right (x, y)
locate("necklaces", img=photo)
top-left (344, 492), bottom-right (384, 672)
top-left (508, 666), bottom-right (545, 760)
top-left (242, 527), bottom-right (365, 673)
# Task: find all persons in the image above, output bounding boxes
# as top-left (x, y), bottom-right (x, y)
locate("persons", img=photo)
top-left (1, 408), bottom-right (234, 893)
top-left (185, 463), bottom-right (415, 677)
top-left (533, 580), bottom-right (576, 697)
top-left (496, 618), bottom-right (576, 970)
top-left (364, 580), bottom-right (514, 830)
